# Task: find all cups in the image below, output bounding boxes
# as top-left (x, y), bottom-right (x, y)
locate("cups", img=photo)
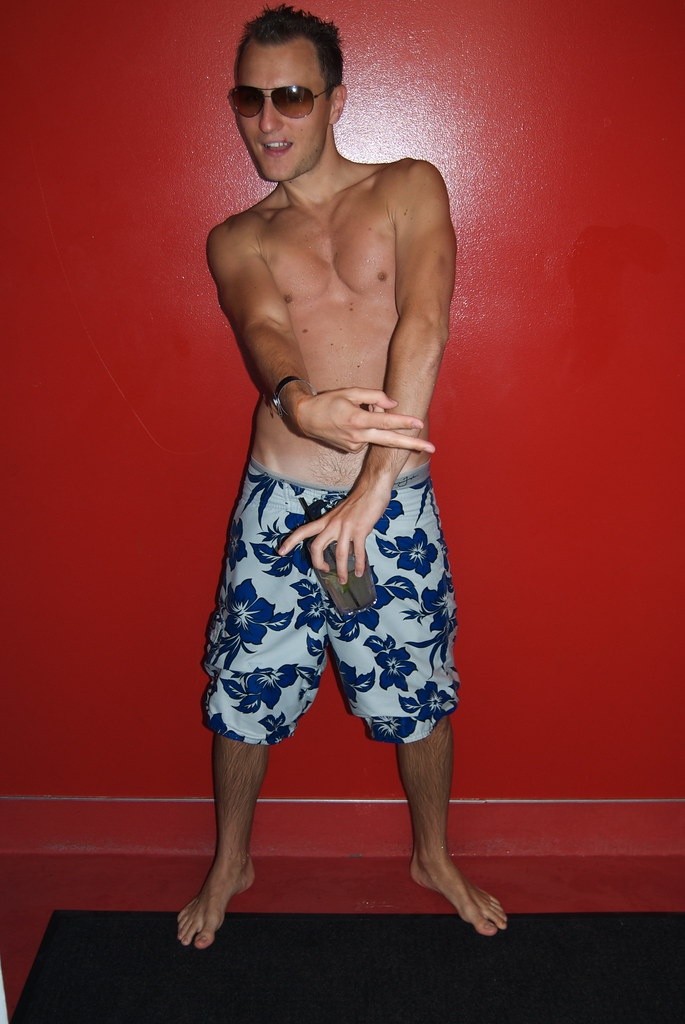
top-left (307, 536), bottom-right (377, 615)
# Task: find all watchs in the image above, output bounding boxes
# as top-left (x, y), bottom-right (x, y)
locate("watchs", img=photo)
top-left (270, 376), bottom-right (316, 417)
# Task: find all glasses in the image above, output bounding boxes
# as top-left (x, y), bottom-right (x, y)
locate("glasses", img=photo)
top-left (227, 85), bottom-right (333, 119)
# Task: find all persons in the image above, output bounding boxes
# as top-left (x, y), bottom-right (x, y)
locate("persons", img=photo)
top-left (178, 5), bottom-right (508, 949)
